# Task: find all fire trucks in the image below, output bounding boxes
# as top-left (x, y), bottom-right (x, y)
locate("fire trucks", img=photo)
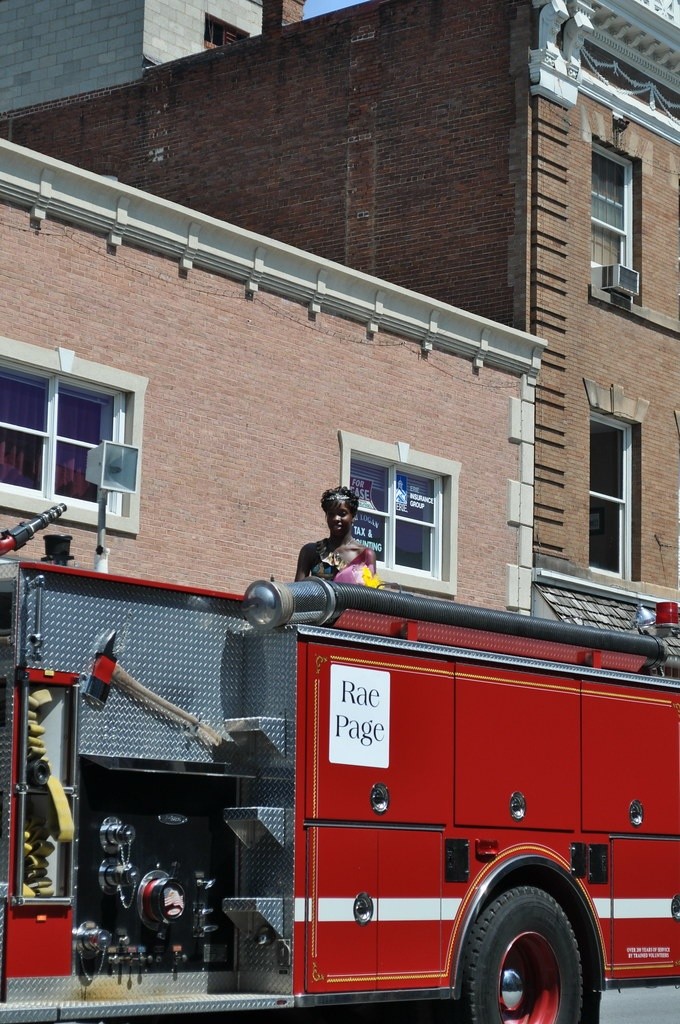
top-left (0, 504), bottom-right (680, 1024)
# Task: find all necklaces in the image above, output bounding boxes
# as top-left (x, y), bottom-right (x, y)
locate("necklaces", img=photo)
top-left (327, 537), bottom-right (354, 556)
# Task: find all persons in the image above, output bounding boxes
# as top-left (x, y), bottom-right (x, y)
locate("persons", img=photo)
top-left (294, 485), bottom-right (377, 592)
